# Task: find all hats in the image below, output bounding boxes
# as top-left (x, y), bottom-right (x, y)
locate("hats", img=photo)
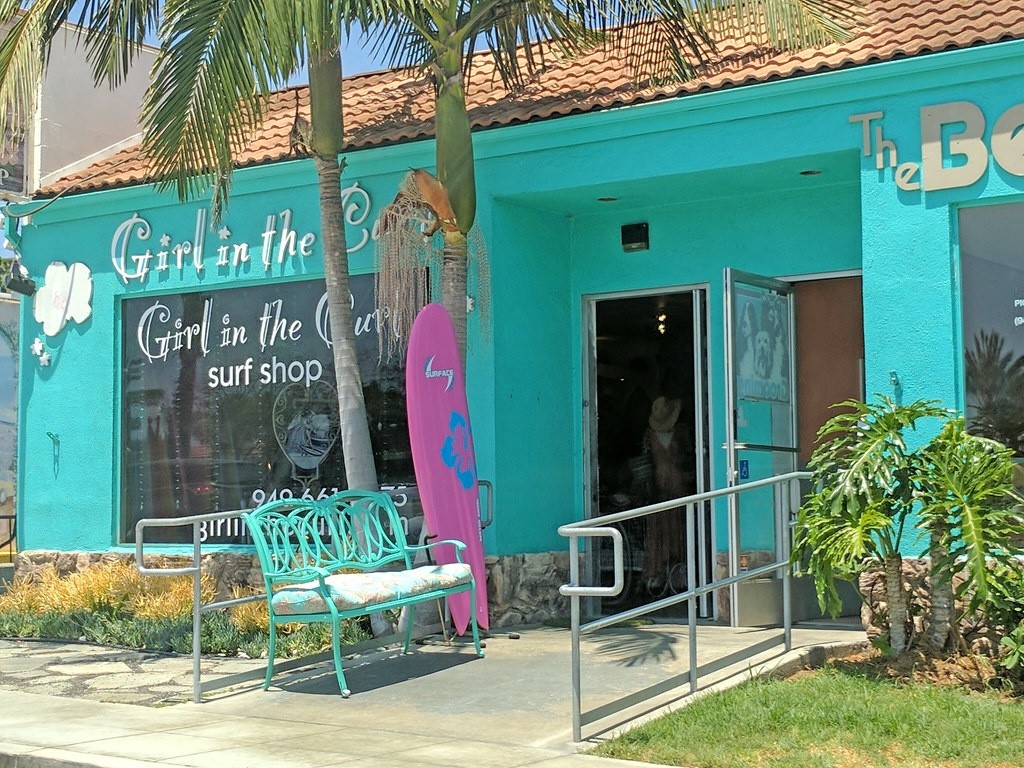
top-left (648, 396), bottom-right (685, 433)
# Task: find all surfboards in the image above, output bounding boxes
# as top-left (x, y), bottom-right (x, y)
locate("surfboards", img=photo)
top-left (406, 302), bottom-right (490, 636)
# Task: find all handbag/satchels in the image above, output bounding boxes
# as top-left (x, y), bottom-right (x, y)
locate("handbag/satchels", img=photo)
top-left (628, 434), bottom-right (653, 498)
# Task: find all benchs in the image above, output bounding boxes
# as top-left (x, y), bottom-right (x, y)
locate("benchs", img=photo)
top-left (241, 490), bottom-right (486, 700)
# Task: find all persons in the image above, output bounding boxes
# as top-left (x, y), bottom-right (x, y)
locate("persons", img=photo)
top-left (640, 397), bottom-right (690, 589)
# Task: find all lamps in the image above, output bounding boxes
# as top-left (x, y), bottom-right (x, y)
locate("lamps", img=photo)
top-left (5, 273), bottom-right (39, 296)
